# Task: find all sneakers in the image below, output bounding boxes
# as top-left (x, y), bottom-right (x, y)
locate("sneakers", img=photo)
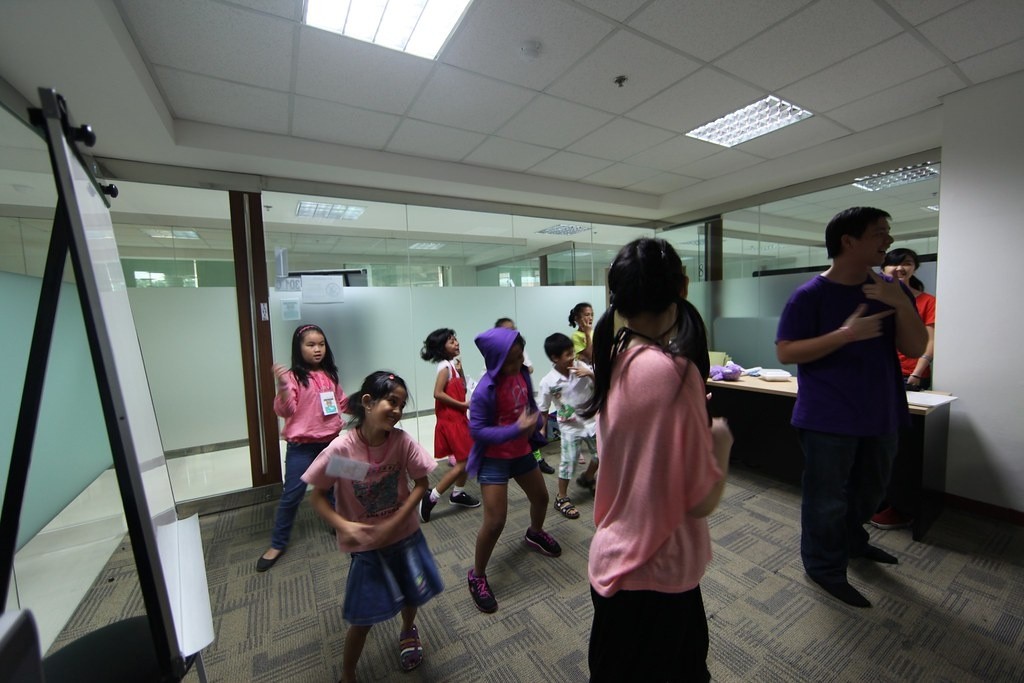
top-left (870, 505), bottom-right (914, 530)
top-left (449, 492), bottom-right (481, 507)
top-left (467, 570), bottom-right (498, 613)
top-left (525, 526), bottom-right (562, 558)
top-left (420, 488), bottom-right (437, 523)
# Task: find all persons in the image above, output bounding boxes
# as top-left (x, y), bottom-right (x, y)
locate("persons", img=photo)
top-left (299, 370), bottom-right (446, 683)
top-left (255, 324), bottom-right (359, 572)
top-left (568, 235), bottom-right (747, 683)
top-left (774, 206), bottom-right (929, 608)
top-left (418, 328), bottom-right (482, 524)
top-left (494, 317), bottom-right (555, 475)
top-left (876, 249), bottom-right (937, 394)
top-left (536, 331), bottom-right (599, 520)
top-left (464, 328), bottom-right (562, 613)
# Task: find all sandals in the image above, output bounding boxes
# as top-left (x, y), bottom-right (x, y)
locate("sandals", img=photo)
top-left (576, 472), bottom-right (596, 496)
top-left (554, 493), bottom-right (580, 520)
top-left (399, 624), bottom-right (423, 671)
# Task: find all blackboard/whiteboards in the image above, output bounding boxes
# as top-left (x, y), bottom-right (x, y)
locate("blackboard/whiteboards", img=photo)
top-left (37, 86), bottom-right (182, 665)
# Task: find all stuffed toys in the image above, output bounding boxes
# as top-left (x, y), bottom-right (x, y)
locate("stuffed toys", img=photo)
top-left (707, 360), bottom-right (744, 382)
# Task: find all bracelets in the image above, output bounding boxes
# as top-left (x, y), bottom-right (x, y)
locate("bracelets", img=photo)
top-left (840, 327), bottom-right (856, 342)
top-left (921, 354), bottom-right (933, 363)
top-left (910, 374), bottom-right (922, 379)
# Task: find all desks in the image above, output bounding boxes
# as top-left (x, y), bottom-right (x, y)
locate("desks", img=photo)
top-left (707, 369), bottom-right (952, 539)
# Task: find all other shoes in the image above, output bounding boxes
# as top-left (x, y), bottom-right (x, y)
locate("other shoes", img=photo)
top-left (539, 459), bottom-right (555, 474)
top-left (255, 548), bottom-right (282, 572)
